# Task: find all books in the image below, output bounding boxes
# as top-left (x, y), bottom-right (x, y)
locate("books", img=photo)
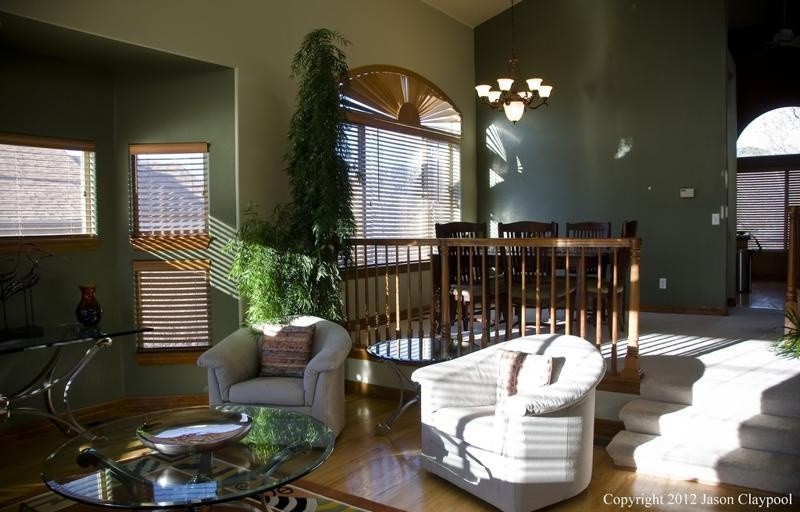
top-left (153, 481), bottom-right (217, 502)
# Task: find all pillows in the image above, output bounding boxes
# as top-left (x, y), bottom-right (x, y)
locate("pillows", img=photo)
top-left (494, 347), bottom-right (554, 407)
top-left (257, 321), bottom-right (317, 380)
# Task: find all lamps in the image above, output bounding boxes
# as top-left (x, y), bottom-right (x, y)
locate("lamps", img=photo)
top-left (473, 1), bottom-right (555, 126)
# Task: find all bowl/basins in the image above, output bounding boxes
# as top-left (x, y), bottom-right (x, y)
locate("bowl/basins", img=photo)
top-left (136, 408), bottom-right (252, 454)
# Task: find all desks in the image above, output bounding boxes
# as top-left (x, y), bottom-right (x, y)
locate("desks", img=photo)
top-left (39, 402), bottom-right (337, 512)
top-left (364, 334), bottom-right (483, 438)
top-left (1, 320), bottom-right (158, 447)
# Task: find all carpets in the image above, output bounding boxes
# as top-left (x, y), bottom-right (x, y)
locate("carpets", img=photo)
top-left (0, 454), bottom-right (406, 512)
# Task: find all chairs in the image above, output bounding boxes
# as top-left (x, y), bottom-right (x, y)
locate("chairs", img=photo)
top-left (195, 313), bottom-right (354, 448)
top-left (427, 219), bottom-right (641, 338)
top-left (410, 332), bottom-right (608, 511)
